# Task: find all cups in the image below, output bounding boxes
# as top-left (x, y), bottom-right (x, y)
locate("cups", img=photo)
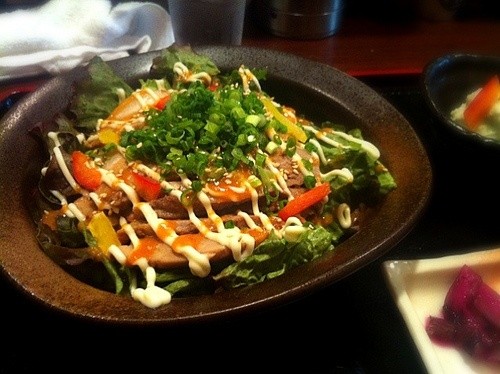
top-left (267, 0), bottom-right (341, 40)
top-left (168, 0), bottom-right (245, 48)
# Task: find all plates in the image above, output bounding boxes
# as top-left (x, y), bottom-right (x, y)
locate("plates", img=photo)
top-left (381, 244), bottom-right (500, 374)
top-left (1, 41), bottom-right (436, 326)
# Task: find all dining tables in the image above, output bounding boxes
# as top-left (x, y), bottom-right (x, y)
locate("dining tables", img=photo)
top-left (0, 69), bottom-right (500, 370)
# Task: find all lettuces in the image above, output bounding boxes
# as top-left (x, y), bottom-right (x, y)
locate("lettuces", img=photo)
top-left (25, 46), bottom-right (397, 296)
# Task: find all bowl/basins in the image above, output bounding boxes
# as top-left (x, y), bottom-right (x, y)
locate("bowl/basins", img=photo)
top-left (423, 49), bottom-right (500, 163)
